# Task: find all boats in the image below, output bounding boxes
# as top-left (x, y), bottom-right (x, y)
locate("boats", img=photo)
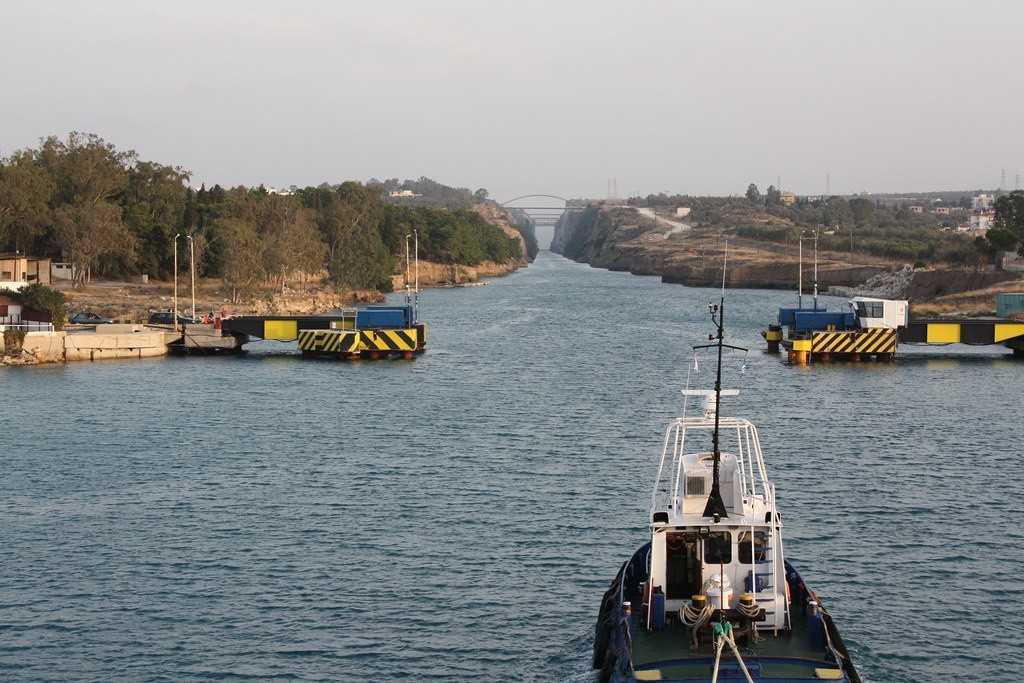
top-left (591, 239), bottom-right (864, 683)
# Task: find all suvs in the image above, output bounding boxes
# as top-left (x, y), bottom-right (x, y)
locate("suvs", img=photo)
top-left (149, 311), bottom-right (195, 325)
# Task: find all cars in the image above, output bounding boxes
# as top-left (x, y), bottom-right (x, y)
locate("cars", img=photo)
top-left (68, 311), bottom-right (114, 324)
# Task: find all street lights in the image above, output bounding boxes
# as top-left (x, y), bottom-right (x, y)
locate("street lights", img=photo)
top-left (405, 234), bottom-right (412, 329)
top-left (798, 230), bottom-right (806, 311)
top-left (186, 235), bottom-right (196, 323)
top-left (413, 229), bottom-right (419, 323)
top-left (173, 233), bottom-right (181, 331)
top-left (811, 229), bottom-right (818, 313)
